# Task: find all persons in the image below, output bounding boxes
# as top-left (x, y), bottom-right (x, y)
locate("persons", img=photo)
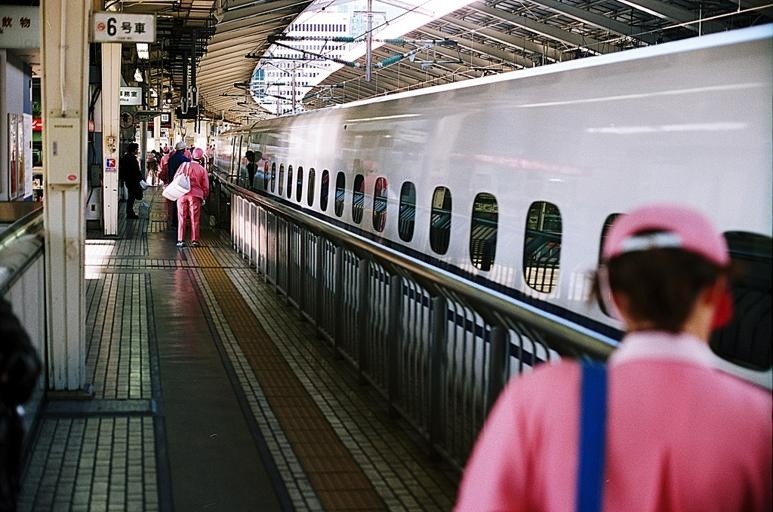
top-left (0, 294), bottom-right (42, 512)
top-left (453, 205), bottom-right (773, 511)
top-left (122, 143), bottom-right (143, 219)
top-left (146, 140), bottom-right (210, 247)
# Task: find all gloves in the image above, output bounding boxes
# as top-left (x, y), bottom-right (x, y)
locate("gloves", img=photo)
top-left (201, 200), bottom-right (205, 206)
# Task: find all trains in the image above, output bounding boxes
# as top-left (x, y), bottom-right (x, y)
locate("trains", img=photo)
top-left (213, 23), bottom-right (773, 414)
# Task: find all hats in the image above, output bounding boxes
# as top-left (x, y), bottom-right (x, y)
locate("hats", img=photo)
top-left (245, 151), bottom-right (252, 157)
top-left (176, 142), bottom-right (186, 150)
top-left (604, 206), bottom-right (732, 328)
top-left (163, 147), bottom-right (168, 153)
top-left (192, 148), bottom-right (203, 160)
top-left (140, 180), bottom-right (147, 190)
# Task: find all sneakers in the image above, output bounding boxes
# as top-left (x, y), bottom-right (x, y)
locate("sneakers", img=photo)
top-left (177, 241), bottom-right (185, 247)
top-left (127, 214), bottom-right (139, 219)
top-left (192, 242), bottom-right (199, 247)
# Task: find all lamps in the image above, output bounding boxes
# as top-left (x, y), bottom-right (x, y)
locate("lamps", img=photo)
top-left (105, 0), bottom-right (124, 13)
top-left (136, 42), bottom-right (149, 60)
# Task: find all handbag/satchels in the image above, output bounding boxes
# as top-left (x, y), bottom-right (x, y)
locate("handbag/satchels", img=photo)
top-left (162, 174), bottom-right (191, 202)
